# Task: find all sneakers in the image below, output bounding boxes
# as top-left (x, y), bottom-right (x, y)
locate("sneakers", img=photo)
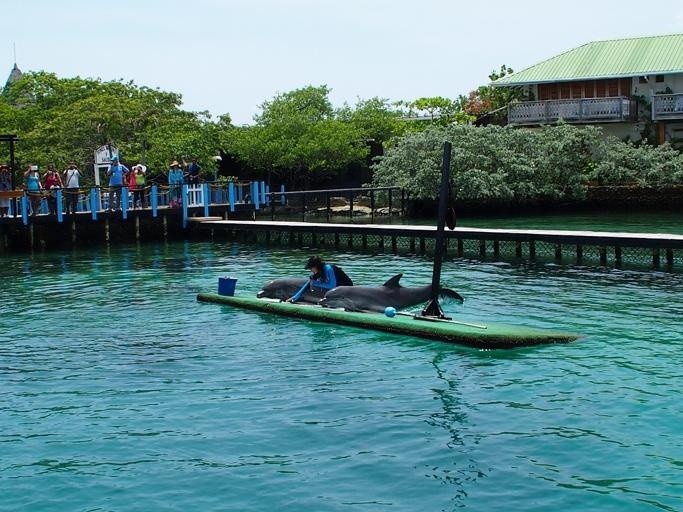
top-left (103, 207), bottom-right (149, 212)
top-left (0, 210), bottom-right (77, 218)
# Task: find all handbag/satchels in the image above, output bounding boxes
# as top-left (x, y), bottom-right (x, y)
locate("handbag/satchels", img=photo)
top-left (170, 199), bottom-right (183, 208)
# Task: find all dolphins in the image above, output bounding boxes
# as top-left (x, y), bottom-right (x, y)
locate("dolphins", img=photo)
top-left (318, 274), bottom-right (464, 313)
top-left (257, 277), bottom-right (326, 303)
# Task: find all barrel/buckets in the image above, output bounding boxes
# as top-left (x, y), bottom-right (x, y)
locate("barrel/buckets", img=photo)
top-left (218, 277), bottom-right (238, 296)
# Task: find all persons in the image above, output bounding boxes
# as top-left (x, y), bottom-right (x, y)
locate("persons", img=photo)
top-left (0, 165), bottom-right (12, 217)
top-left (131, 163), bottom-right (147, 209)
top-left (168, 161), bottom-right (184, 208)
top-left (104, 156), bottom-right (130, 212)
top-left (189, 158), bottom-right (201, 203)
top-left (286, 255), bottom-right (353, 304)
top-left (24, 160), bottom-right (82, 215)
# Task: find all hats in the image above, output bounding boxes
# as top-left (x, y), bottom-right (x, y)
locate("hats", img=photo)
top-left (303, 255), bottom-right (322, 270)
top-left (110, 155), bottom-right (120, 161)
top-left (169, 160), bottom-right (182, 168)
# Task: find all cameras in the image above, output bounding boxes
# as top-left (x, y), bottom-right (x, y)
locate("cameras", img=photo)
top-left (30, 166), bottom-right (37, 171)
top-left (70, 165), bottom-right (74, 170)
